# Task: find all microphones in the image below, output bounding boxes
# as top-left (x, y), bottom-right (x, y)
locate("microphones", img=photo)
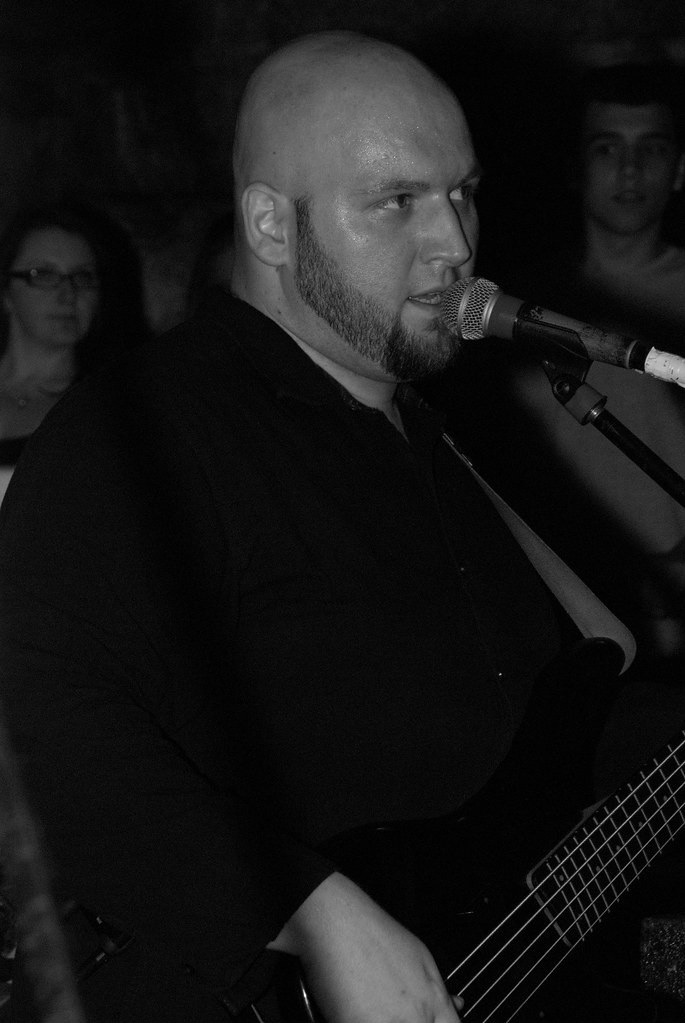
top-left (439, 275), bottom-right (685, 388)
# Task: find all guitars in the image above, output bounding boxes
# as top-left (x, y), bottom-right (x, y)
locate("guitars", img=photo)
top-left (227, 721), bottom-right (685, 1023)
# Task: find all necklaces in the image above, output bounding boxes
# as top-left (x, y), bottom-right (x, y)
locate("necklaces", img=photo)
top-left (6, 390), bottom-right (32, 411)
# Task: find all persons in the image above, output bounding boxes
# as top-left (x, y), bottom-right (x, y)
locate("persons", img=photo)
top-left (0, 30), bottom-right (685, 1023)
top-left (0, 212), bottom-right (102, 467)
top-left (576, 64), bottom-right (685, 320)
